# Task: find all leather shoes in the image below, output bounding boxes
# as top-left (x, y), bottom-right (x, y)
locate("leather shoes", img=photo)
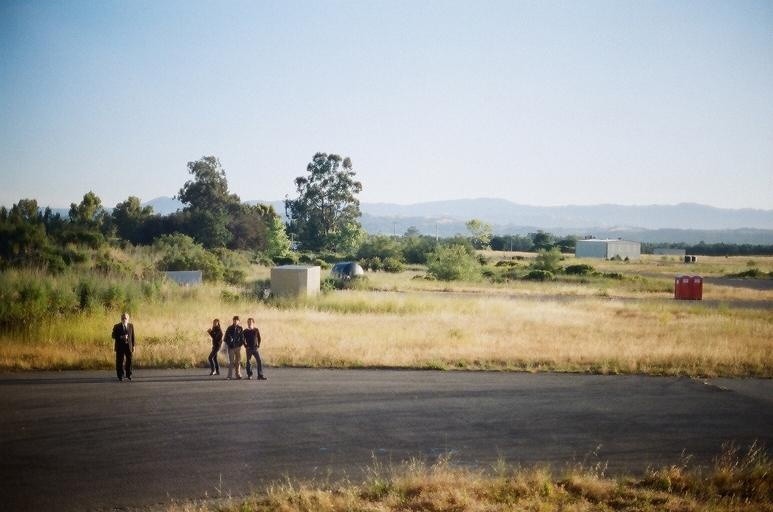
top-left (236, 376), bottom-right (243, 379)
top-left (258, 375), bottom-right (267, 380)
top-left (248, 375), bottom-right (252, 380)
top-left (226, 377), bottom-right (232, 380)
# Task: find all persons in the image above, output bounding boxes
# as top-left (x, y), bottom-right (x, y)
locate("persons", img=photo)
top-left (207, 316), bottom-right (267, 380)
top-left (112, 313), bottom-right (134, 381)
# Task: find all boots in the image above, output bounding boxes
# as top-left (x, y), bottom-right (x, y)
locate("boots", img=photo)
top-left (210, 368), bottom-right (220, 376)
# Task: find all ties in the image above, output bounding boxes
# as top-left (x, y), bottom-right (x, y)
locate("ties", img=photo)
top-left (124, 326), bottom-right (127, 335)
